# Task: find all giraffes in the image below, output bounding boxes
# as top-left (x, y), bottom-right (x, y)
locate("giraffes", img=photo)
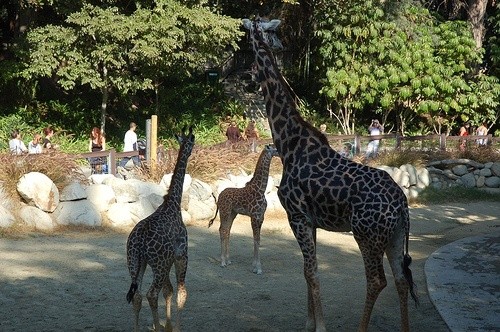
top-left (208, 144), bottom-right (281, 275)
top-left (127, 126), bottom-right (195, 332)
top-left (234, 12), bottom-right (420, 332)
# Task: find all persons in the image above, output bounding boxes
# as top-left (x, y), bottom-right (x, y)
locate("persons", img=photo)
top-left (365, 119), bottom-right (384, 160)
top-left (245, 120), bottom-right (258, 140)
top-left (459, 120), bottom-right (471, 153)
top-left (226, 122), bottom-right (245, 142)
top-left (340, 142), bottom-right (354, 160)
top-left (28, 134), bottom-right (42, 155)
top-left (476, 122), bottom-right (488, 149)
top-left (43, 127), bottom-right (53, 153)
top-left (122, 123), bottom-right (139, 170)
top-left (8, 130), bottom-right (28, 156)
top-left (88, 127), bottom-right (106, 169)
